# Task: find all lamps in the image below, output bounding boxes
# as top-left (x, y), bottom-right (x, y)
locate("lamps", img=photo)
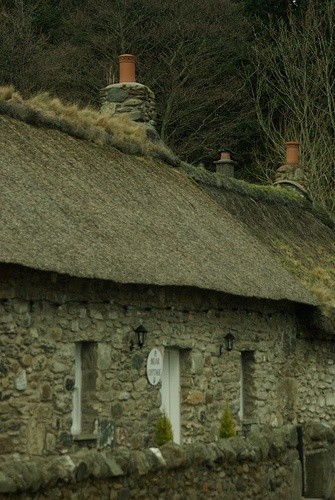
top-left (219, 332), bottom-right (235, 354)
top-left (130, 325), bottom-right (147, 350)
